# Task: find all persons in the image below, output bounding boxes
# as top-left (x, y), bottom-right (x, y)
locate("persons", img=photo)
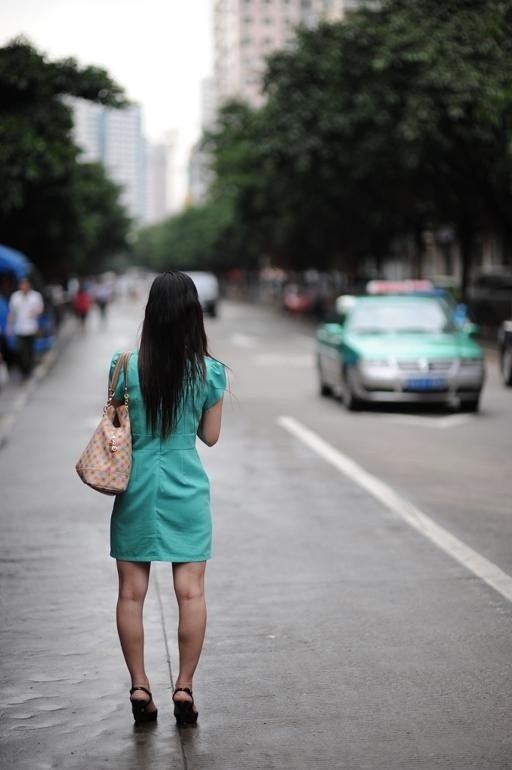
top-left (77, 272), bottom-right (230, 728)
top-left (95, 275), bottom-right (114, 317)
top-left (5, 274), bottom-right (45, 381)
top-left (71, 283), bottom-right (91, 326)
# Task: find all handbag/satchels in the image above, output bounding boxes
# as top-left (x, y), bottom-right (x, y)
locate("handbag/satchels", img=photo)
top-left (74, 349), bottom-right (135, 496)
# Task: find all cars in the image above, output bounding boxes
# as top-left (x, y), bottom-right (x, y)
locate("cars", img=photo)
top-left (181, 270), bottom-right (221, 317)
top-left (283, 284), bottom-right (320, 314)
top-left (498, 317), bottom-right (512, 386)
top-left (314, 280), bottom-right (486, 414)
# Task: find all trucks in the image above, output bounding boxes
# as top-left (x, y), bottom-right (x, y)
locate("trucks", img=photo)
top-left (0, 246), bottom-right (55, 365)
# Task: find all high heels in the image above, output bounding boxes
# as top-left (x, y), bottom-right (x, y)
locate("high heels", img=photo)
top-left (171, 687), bottom-right (198, 729)
top-left (128, 686), bottom-right (158, 727)
